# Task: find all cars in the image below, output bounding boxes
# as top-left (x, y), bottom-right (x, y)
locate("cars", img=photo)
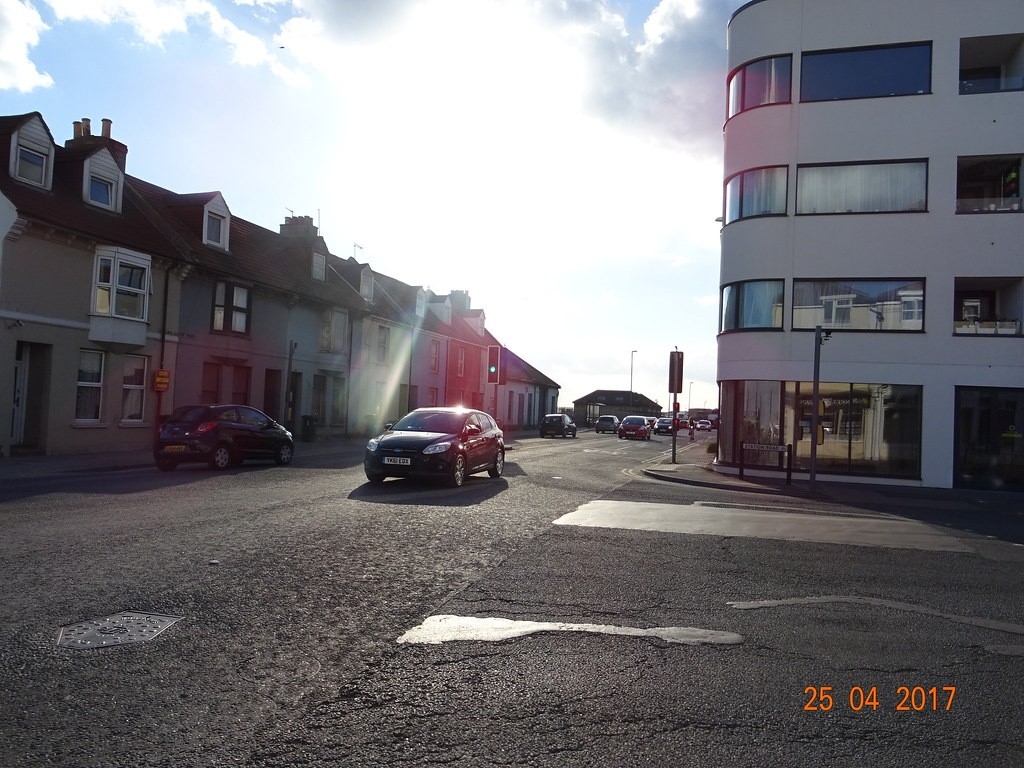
top-left (654, 418), bottom-right (673, 436)
top-left (647, 416), bottom-right (658, 430)
top-left (696, 420), bottom-right (712, 431)
top-left (364, 407), bottom-right (506, 487)
top-left (595, 415), bottom-right (621, 433)
top-left (618, 415), bottom-right (651, 440)
top-left (540, 413), bottom-right (577, 438)
top-left (680, 420), bottom-right (698, 429)
top-left (154, 402), bottom-right (295, 472)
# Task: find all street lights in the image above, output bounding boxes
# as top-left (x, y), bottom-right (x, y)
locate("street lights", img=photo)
top-left (688, 382), bottom-right (693, 409)
top-left (630, 350), bottom-right (638, 413)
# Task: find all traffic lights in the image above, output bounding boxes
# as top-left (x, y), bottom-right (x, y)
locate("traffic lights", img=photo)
top-left (673, 403), bottom-right (680, 413)
top-left (487, 345), bottom-right (501, 384)
top-left (673, 419), bottom-right (680, 431)
